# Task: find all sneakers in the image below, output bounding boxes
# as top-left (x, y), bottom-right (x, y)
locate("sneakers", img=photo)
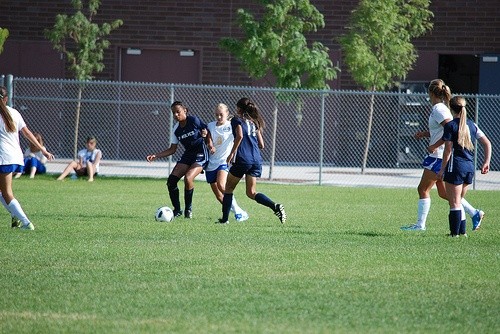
top-left (214, 219), bottom-right (230, 225)
top-left (401, 224), bottom-right (426, 231)
top-left (273, 203), bottom-right (287, 224)
top-left (471, 210), bottom-right (485, 231)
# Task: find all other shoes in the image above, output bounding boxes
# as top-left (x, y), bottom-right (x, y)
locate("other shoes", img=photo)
top-left (10, 218), bottom-right (34, 230)
top-left (184, 210), bottom-right (192, 218)
top-left (445, 232), bottom-right (468, 238)
top-left (235, 212), bottom-right (250, 222)
top-left (172, 208), bottom-right (183, 217)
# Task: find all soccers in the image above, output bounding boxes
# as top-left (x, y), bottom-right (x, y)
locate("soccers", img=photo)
top-left (155, 206), bottom-right (174, 222)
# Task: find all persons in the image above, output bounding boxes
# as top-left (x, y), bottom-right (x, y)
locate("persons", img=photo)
top-left (16, 134), bottom-right (46, 179)
top-left (202, 103), bottom-right (249, 221)
top-left (401, 79), bottom-right (485, 231)
top-left (146, 101), bottom-right (217, 218)
top-left (56, 137), bottom-right (102, 182)
top-left (0, 85), bottom-right (55, 229)
top-left (214, 97), bottom-right (287, 225)
top-left (437, 96), bottom-right (492, 237)
